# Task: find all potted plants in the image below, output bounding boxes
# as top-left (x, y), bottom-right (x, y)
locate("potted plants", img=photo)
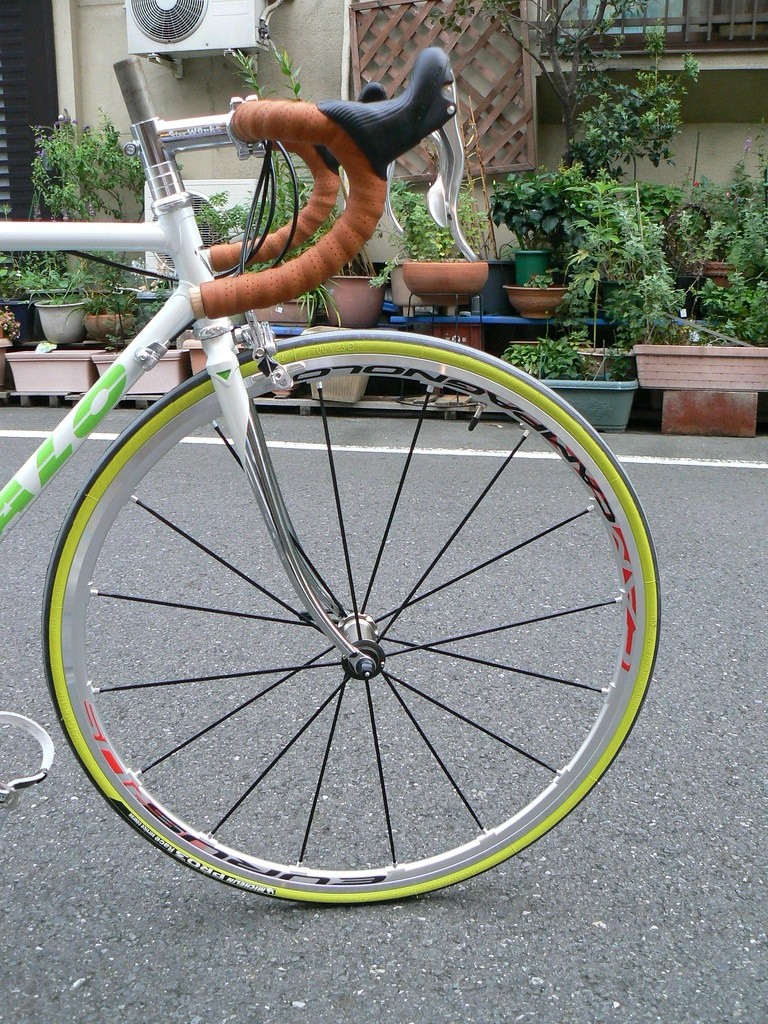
top-left (0, 107), bottom-right (768, 435)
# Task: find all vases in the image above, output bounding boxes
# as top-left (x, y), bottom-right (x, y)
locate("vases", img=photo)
top-left (0, 338), bottom-right (14, 391)
top-left (4, 349), bottom-right (106, 395)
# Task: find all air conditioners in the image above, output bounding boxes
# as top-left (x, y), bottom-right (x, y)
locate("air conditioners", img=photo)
top-left (125, 0), bottom-right (269, 79)
top-left (145, 177), bottom-right (274, 281)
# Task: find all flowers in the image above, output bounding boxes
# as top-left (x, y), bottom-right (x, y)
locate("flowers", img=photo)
top-left (27, 108), bottom-right (151, 221)
top-left (0, 306), bottom-right (21, 339)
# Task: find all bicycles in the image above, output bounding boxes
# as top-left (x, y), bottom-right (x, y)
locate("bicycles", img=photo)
top-left (0, 52), bottom-right (664, 906)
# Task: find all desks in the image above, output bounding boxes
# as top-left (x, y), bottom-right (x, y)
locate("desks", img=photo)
top-left (390, 315), bottom-right (719, 396)
